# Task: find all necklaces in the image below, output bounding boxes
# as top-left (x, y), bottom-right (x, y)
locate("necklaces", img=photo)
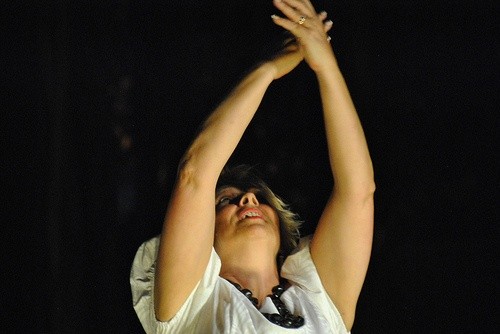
top-left (227, 279), bottom-right (305, 329)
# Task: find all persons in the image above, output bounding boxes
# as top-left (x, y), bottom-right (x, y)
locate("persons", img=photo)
top-left (130, 0), bottom-right (376, 334)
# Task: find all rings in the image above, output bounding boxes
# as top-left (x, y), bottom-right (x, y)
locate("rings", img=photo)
top-left (298, 15), bottom-right (306, 25)
